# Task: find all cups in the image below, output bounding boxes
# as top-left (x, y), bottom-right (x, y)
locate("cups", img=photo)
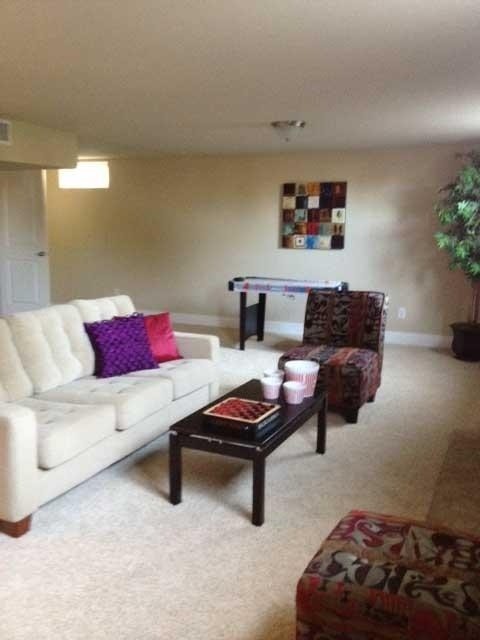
top-left (282, 380), bottom-right (307, 405)
top-left (259, 376), bottom-right (284, 400)
top-left (264, 368), bottom-right (286, 383)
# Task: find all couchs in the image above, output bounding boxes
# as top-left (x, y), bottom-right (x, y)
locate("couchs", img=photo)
top-left (1, 292), bottom-right (222, 541)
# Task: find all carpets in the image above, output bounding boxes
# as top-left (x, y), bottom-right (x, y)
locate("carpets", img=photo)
top-left (423, 427), bottom-right (480, 538)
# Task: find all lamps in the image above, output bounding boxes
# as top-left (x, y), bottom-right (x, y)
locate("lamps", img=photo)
top-left (268, 117), bottom-right (307, 143)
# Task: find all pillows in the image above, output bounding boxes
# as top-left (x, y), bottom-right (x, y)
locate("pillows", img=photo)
top-left (80, 312), bottom-right (161, 378)
top-left (112, 307), bottom-right (181, 364)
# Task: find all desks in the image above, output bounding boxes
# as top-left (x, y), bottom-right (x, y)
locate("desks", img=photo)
top-left (227, 274), bottom-right (350, 353)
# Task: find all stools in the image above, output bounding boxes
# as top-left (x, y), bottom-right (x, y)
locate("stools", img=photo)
top-left (294, 510), bottom-right (480, 639)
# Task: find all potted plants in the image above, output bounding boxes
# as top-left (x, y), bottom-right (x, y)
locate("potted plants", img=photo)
top-left (432, 149), bottom-right (480, 364)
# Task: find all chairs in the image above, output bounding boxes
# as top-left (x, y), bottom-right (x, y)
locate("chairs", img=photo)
top-left (274, 286), bottom-right (390, 426)
